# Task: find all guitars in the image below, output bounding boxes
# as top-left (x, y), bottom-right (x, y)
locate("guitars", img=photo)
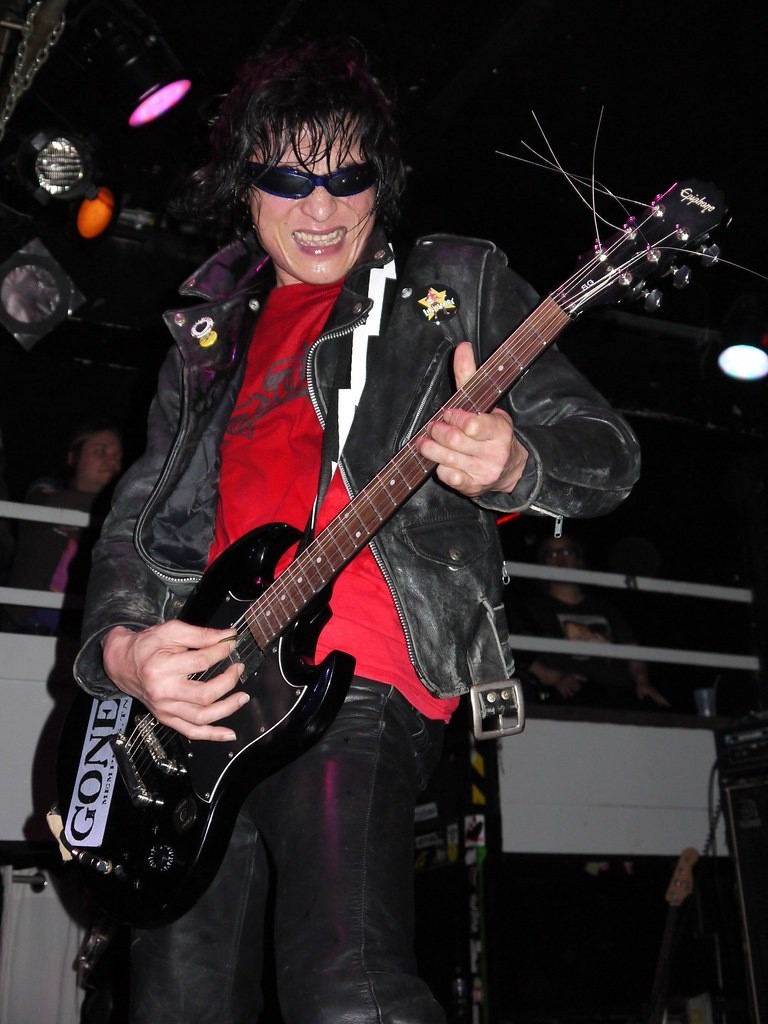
top-left (56, 174), bottom-right (736, 928)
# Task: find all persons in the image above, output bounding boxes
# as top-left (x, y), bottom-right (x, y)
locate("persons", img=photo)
top-left (71, 41), bottom-right (642, 1024)
top-left (0, 418), bottom-right (123, 635)
top-left (511, 535), bottom-right (670, 707)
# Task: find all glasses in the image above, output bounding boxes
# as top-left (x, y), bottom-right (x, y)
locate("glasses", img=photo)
top-left (234, 157), bottom-right (381, 199)
